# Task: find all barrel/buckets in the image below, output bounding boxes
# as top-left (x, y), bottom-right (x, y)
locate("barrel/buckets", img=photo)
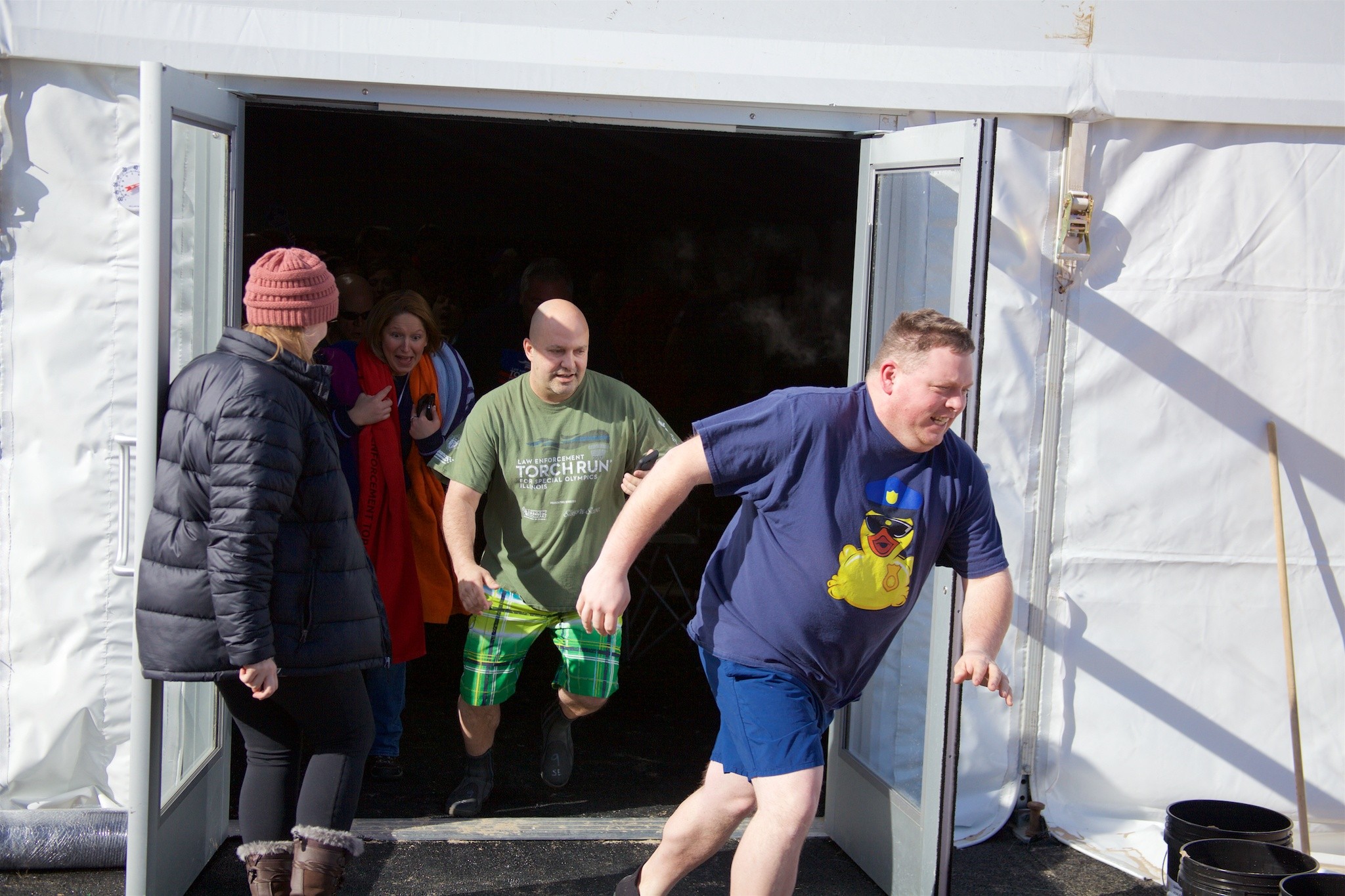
top-left (1161, 799), bottom-right (1295, 896)
top-left (1176, 838), bottom-right (1320, 896)
top-left (1277, 873), bottom-right (1345, 896)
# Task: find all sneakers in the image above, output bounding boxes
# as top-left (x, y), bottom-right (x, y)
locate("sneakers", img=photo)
top-left (368, 755), bottom-right (404, 777)
top-left (445, 767), bottom-right (494, 816)
top-left (539, 703), bottom-right (574, 789)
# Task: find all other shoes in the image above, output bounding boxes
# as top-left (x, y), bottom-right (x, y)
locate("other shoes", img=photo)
top-left (612, 860), bottom-right (645, 896)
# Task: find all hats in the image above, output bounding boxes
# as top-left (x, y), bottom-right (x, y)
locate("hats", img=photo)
top-left (241, 247), bottom-right (338, 327)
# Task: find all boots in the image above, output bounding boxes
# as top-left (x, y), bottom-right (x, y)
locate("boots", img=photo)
top-left (237, 840), bottom-right (293, 896)
top-left (287, 825), bottom-right (364, 896)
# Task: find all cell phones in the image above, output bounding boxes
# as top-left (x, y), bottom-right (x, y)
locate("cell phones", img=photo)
top-left (633, 449), bottom-right (659, 476)
top-left (417, 393), bottom-right (435, 421)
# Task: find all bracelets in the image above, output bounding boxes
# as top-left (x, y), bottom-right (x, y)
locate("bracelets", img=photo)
top-left (410, 415), bottom-right (417, 421)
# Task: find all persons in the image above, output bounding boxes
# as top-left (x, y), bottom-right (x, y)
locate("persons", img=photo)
top-left (425, 298), bottom-right (685, 815)
top-left (575, 308), bottom-right (1013, 896)
top-left (304, 228), bottom-right (461, 348)
top-left (318, 293), bottom-right (475, 801)
top-left (461, 253), bottom-right (852, 395)
top-left (135, 247), bottom-right (394, 896)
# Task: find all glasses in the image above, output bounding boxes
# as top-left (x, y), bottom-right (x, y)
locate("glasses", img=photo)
top-left (341, 310), bottom-right (370, 321)
